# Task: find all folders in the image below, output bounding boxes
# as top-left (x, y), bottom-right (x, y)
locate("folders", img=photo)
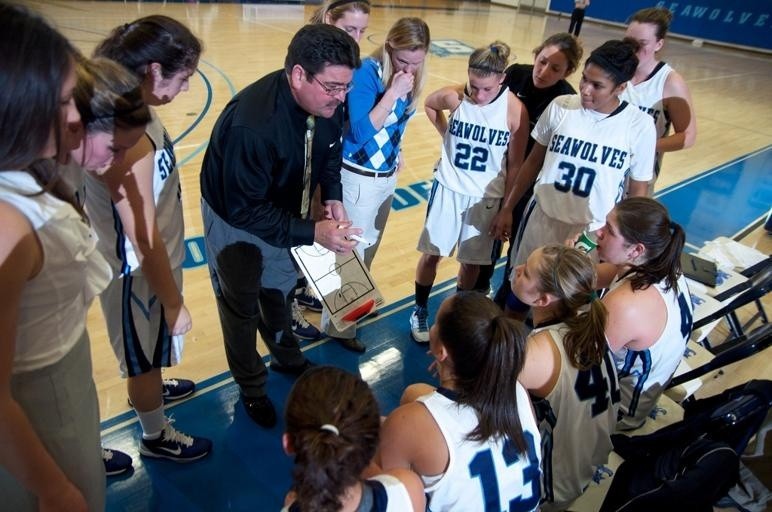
top-left (292, 225), bottom-right (386, 333)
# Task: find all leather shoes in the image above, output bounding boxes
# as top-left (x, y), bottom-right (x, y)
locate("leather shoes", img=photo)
top-left (334, 336), bottom-right (367, 352)
top-left (240, 390), bottom-right (276, 427)
top-left (269, 355), bottom-right (318, 373)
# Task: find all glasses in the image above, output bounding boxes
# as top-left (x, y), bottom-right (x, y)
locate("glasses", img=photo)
top-left (311, 73), bottom-right (354, 96)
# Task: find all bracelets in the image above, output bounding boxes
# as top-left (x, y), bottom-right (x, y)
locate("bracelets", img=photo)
top-left (378, 102), bottom-right (392, 113)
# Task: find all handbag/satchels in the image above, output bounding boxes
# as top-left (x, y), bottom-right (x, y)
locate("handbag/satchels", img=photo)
top-left (682, 378), bottom-right (770, 462)
top-left (599, 436), bottom-right (739, 512)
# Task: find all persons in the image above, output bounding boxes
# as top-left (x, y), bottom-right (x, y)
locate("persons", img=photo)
top-left (44, 40), bottom-right (154, 476)
top-left (473, 32), bottom-right (583, 310)
top-left (564, 197), bottom-right (693, 432)
top-left (86, 15), bottom-right (213, 461)
top-left (494, 36), bottom-right (657, 322)
top-left (322, 18), bottom-right (430, 352)
top-left (568, 0), bottom-right (590, 36)
top-left (200, 23), bottom-right (364, 429)
top-left (0, 0), bottom-right (113, 512)
top-left (280, 365), bottom-right (426, 512)
top-left (361, 291), bottom-right (544, 512)
top-left (288, 0), bottom-right (370, 340)
top-left (618, 7), bottom-right (697, 201)
top-left (426, 243), bottom-right (617, 512)
top-left (409, 40), bottom-right (528, 342)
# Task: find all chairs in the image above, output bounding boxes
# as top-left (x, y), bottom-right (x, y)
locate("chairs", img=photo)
top-left (678, 238), bottom-right (772, 381)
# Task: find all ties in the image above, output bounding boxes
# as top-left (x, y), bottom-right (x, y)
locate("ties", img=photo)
top-left (300, 117), bottom-right (314, 220)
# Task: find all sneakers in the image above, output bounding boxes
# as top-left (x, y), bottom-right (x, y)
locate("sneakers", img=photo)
top-left (294, 287), bottom-right (324, 312)
top-left (127, 379), bottom-right (195, 406)
top-left (288, 302), bottom-right (321, 338)
top-left (101, 448), bottom-right (131, 477)
top-left (139, 426), bottom-right (211, 463)
top-left (410, 308), bottom-right (430, 342)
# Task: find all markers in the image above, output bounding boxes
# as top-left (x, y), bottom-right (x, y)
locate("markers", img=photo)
top-left (336, 225), bottom-right (371, 245)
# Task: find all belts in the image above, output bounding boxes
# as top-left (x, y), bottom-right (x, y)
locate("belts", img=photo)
top-left (341, 163), bottom-right (397, 178)
